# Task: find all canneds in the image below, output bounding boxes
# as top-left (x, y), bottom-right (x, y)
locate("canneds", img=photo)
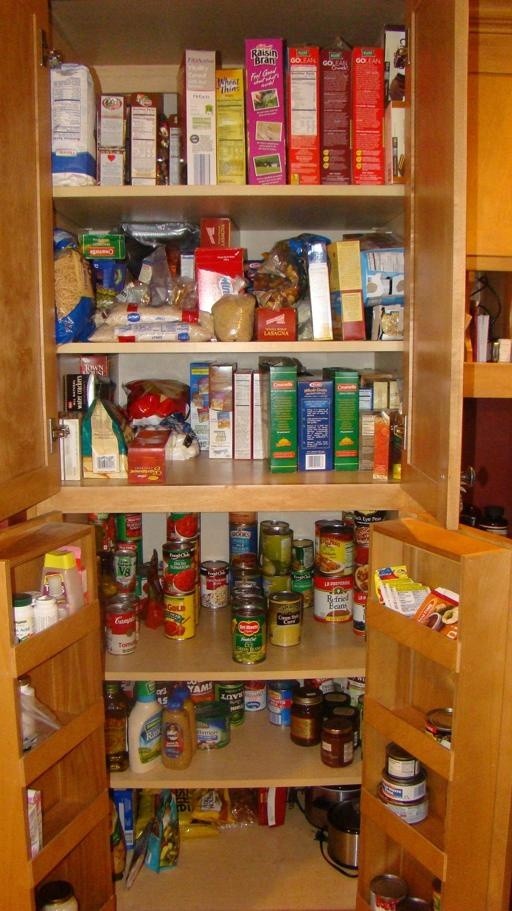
top-left (343, 512), bottom-right (384, 565)
top-left (293, 571), bottom-right (312, 609)
top-left (318, 692), bottom-right (363, 767)
top-left (288, 687), bottom-right (322, 746)
top-left (245, 681), bottom-right (265, 711)
top-left (164, 597), bottom-right (198, 640)
top-left (232, 559), bottom-right (263, 663)
top-left (261, 526), bottom-right (292, 574)
top-left (261, 519), bottom-right (290, 530)
top-left (264, 577), bottom-right (291, 595)
top-left (166, 512), bottom-right (198, 542)
top-left (266, 683), bottom-right (298, 725)
top-left (115, 512), bottom-right (144, 541)
top-left (315, 519), bottom-right (357, 579)
top-left (271, 591), bottom-right (303, 648)
top-left (313, 575), bottom-right (353, 624)
top-left (120, 538), bottom-right (142, 565)
top-left (163, 542), bottom-right (200, 596)
top-left (293, 540), bottom-right (314, 571)
top-left (352, 591), bottom-right (370, 635)
top-left (215, 683), bottom-right (246, 726)
top-left (105, 605), bottom-right (138, 657)
top-left (112, 551), bottom-right (136, 595)
top-left (40, 880), bottom-right (80, 910)
top-left (199, 561), bottom-right (229, 612)
top-left (370, 875), bottom-right (406, 910)
top-left (378, 743), bottom-right (429, 824)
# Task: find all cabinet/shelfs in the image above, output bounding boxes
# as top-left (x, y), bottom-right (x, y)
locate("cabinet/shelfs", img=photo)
top-left (1, 0), bottom-right (471, 534)
top-left (1, 508), bottom-right (512, 911)
top-left (462, 2), bottom-right (512, 402)
